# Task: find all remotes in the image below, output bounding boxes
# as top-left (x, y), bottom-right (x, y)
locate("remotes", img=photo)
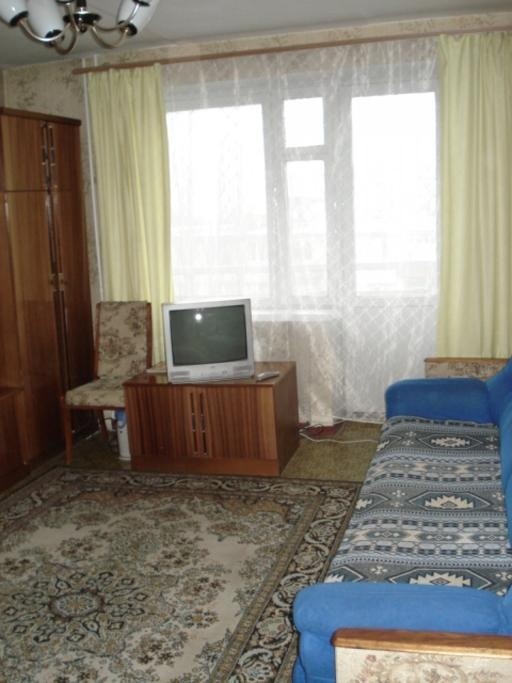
top-left (257, 370), bottom-right (280, 381)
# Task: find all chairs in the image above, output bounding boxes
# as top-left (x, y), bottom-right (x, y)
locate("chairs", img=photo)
top-left (59, 300), bottom-right (153, 465)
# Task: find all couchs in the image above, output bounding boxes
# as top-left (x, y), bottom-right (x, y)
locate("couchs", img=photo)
top-left (294, 356), bottom-right (512, 683)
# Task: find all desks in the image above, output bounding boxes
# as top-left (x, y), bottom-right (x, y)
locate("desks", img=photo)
top-left (123, 361), bottom-right (301, 477)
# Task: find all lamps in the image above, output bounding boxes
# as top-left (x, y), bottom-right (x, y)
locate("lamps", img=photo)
top-left (0, 0), bottom-right (160, 55)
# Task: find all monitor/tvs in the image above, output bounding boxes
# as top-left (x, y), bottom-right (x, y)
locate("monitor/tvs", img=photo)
top-left (161, 299), bottom-right (255, 385)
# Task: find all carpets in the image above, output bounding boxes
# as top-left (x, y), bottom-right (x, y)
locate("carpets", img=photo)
top-left (0, 464), bottom-right (364, 683)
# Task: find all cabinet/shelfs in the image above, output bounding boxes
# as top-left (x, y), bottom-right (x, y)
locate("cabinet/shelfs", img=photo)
top-left (0, 107), bottom-right (104, 492)
